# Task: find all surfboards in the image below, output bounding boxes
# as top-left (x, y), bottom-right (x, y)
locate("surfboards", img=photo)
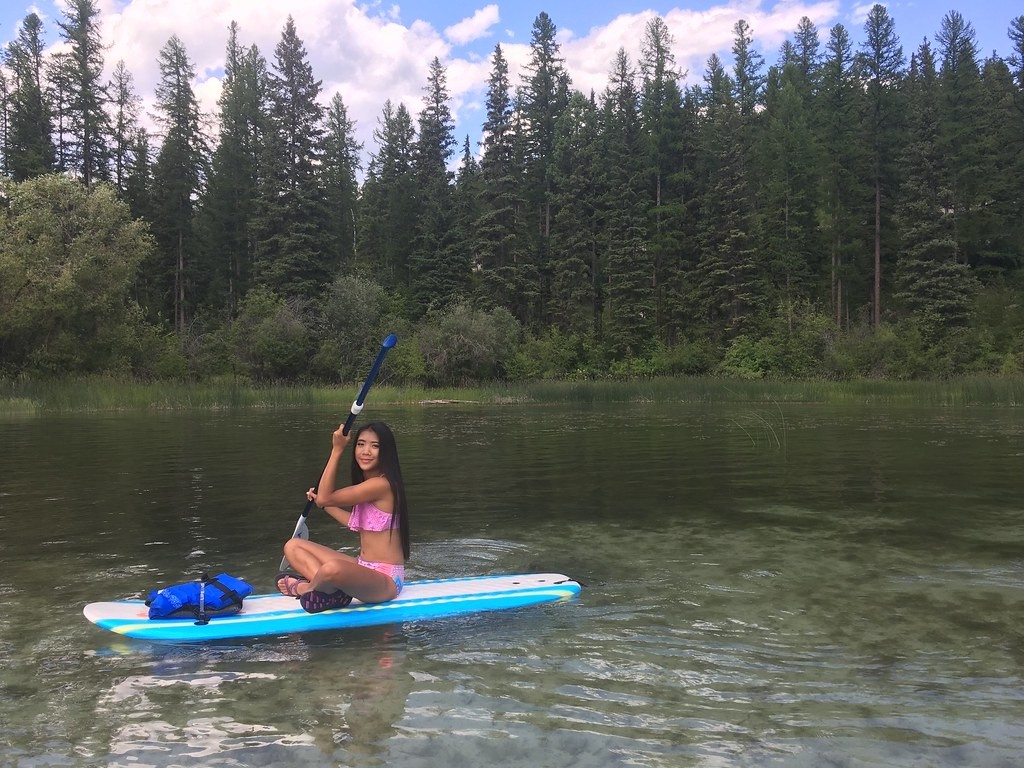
top-left (82, 573), bottom-right (581, 641)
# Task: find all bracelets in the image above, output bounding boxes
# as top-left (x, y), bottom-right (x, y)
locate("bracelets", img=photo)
top-left (322, 506), bottom-right (325, 510)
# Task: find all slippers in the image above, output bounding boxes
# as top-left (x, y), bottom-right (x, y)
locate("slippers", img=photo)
top-left (274, 573), bottom-right (309, 596)
top-left (300, 590), bottom-right (352, 613)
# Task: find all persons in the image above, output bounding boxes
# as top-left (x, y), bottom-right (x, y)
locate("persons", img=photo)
top-left (275, 422), bottom-right (410, 613)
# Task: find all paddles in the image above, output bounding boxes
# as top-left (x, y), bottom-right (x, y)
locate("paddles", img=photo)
top-left (279, 334), bottom-right (398, 571)
